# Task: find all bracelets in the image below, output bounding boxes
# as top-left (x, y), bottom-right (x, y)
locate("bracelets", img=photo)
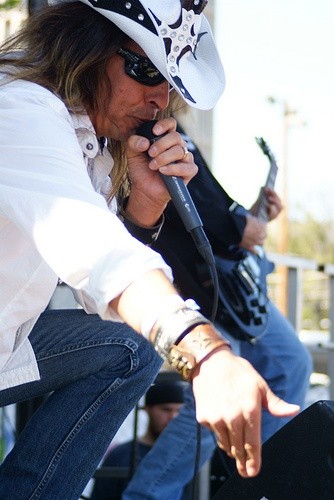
top-left (116, 196), bottom-right (165, 245)
top-left (148, 307), bottom-right (211, 360)
top-left (168, 324), bottom-right (231, 380)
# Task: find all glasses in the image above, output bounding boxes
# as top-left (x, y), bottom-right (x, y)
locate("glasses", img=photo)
top-left (108, 43), bottom-right (168, 87)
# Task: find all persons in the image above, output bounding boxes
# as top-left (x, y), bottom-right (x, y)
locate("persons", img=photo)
top-left (0, 0), bottom-right (300, 500)
top-left (121, 115), bottom-right (314, 500)
top-left (90, 372), bottom-right (187, 500)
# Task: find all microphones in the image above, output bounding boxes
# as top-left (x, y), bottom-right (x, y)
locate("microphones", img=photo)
top-left (136, 120), bottom-right (215, 266)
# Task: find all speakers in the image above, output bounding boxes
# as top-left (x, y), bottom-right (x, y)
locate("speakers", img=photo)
top-left (213, 400), bottom-right (334, 500)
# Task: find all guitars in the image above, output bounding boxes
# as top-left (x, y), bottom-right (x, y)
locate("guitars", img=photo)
top-left (214, 137), bottom-right (279, 342)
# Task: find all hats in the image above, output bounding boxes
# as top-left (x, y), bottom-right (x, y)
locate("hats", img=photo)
top-left (47, 0), bottom-right (226, 112)
top-left (145, 370), bottom-right (192, 406)
